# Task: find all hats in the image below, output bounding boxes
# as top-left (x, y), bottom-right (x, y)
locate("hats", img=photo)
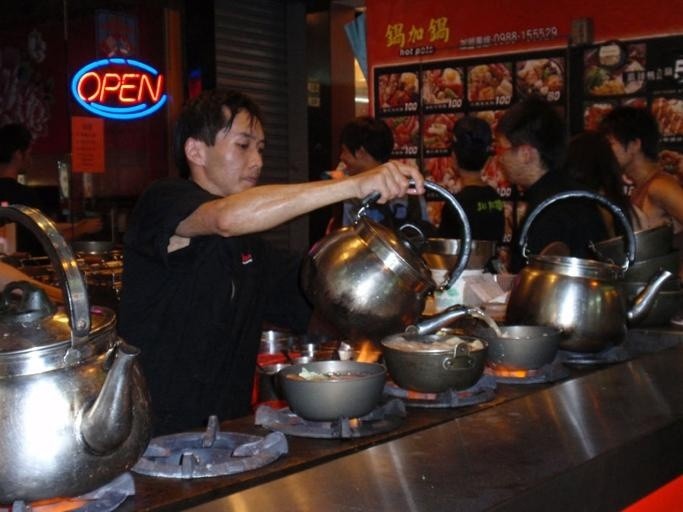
top-left (445, 117), bottom-right (493, 149)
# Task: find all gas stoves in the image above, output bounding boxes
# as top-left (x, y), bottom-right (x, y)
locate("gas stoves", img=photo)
top-left (0, 358), bottom-right (682, 512)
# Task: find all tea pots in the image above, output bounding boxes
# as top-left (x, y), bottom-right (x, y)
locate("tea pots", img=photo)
top-left (300, 179), bottom-right (474, 338)
top-left (503, 190), bottom-right (671, 362)
top-left (1, 203), bottom-right (152, 501)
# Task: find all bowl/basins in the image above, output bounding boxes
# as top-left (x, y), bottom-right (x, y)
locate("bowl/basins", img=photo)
top-left (414, 233), bottom-right (491, 272)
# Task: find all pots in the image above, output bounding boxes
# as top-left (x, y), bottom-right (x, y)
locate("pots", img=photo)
top-left (282, 327), bottom-right (551, 418)
top-left (594, 221), bottom-right (682, 328)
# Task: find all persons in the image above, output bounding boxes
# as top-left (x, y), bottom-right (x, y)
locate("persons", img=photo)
top-left (600, 106), bottom-right (683, 286)
top-left (115, 87), bottom-right (427, 439)
top-left (553, 128), bottom-right (649, 240)
top-left (420, 115), bottom-right (505, 243)
top-left (324, 115), bottom-right (429, 230)
top-left (0, 122), bottom-right (104, 258)
top-left (494, 93), bottom-right (617, 275)
